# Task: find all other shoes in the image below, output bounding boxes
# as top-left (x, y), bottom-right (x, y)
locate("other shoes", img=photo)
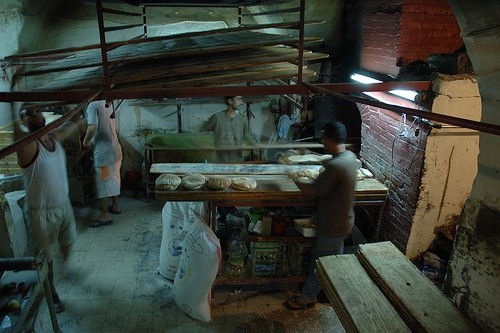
top-left (52, 293), bottom-right (65, 312)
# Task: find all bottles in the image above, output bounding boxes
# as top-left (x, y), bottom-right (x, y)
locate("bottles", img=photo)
top-left (222, 234), bottom-right (247, 280)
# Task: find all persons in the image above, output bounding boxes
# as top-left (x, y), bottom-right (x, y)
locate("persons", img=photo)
top-left (81, 95), bottom-right (124, 226)
top-left (198, 93), bottom-right (258, 165)
top-left (287, 121), bottom-right (357, 309)
top-left (11, 68), bottom-right (81, 312)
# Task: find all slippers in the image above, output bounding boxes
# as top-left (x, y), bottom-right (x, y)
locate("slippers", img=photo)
top-left (100, 205), bottom-right (122, 214)
top-left (282, 295), bottom-right (316, 309)
top-left (90, 219), bottom-right (113, 228)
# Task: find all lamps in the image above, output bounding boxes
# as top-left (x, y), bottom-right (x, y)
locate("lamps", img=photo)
top-left (349, 71), bottom-right (424, 104)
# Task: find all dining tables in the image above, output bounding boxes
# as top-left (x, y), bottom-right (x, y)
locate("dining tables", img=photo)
top-left (146, 160), bottom-right (389, 298)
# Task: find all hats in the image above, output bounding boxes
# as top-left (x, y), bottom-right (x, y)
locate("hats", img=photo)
top-left (324, 121), bottom-right (347, 142)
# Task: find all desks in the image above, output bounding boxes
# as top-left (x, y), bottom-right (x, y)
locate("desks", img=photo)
top-left (0, 248), bottom-right (62, 333)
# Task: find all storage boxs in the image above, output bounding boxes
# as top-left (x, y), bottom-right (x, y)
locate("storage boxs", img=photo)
top-left (293, 217), bottom-right (321, 237)
